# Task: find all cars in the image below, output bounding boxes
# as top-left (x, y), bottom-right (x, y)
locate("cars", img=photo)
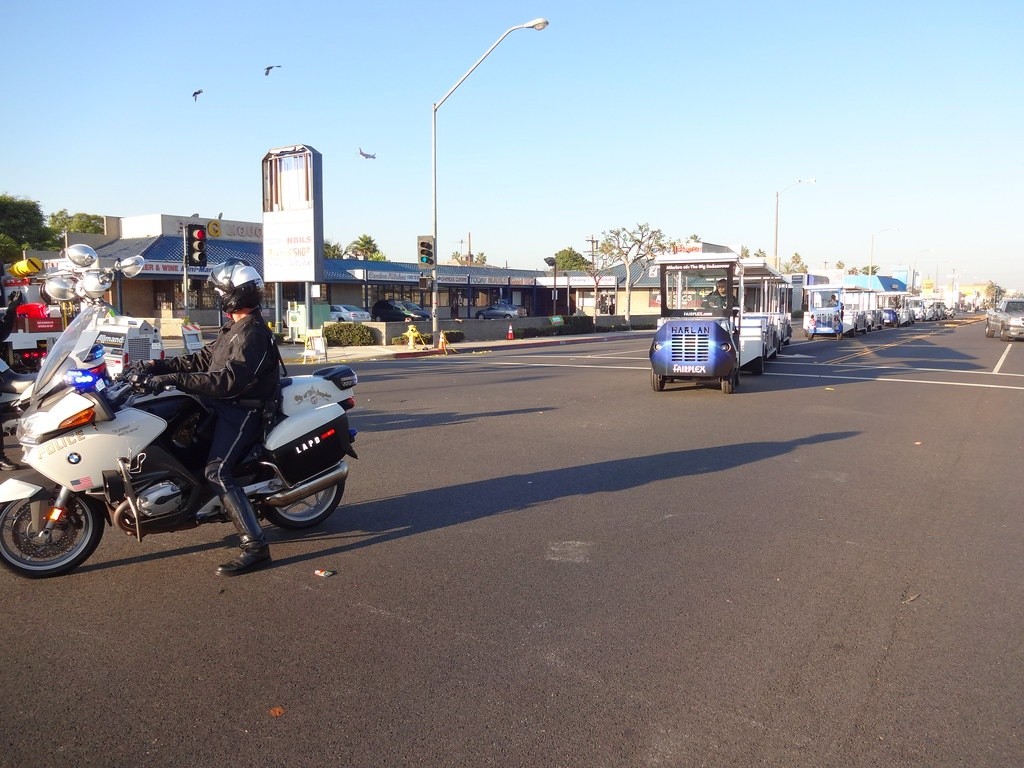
top-left (372, 299), bottom-right (433, 320)
top-left (476, 304), bottom-right (527, 319)
top-left (330, 305), bottom-right (371, 322)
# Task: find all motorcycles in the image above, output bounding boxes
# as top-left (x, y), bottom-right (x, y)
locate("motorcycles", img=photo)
top-left (0, 304), bottom-right (365, 576)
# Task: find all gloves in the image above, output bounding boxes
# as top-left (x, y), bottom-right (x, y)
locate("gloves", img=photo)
top-left (129, 359), bottom-right (150, 376)
top-left (141, 372), bottom-right (177, 396)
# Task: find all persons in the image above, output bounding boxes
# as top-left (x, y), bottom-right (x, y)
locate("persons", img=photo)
top-left (137, 258), bottom-right (280, 576)
top-left (609, 304), bottom-right (614, 315)
top-left (0, 290), bottom-right (23, 471)
top-left (701, 279), bottom-right (738, 315)
top-left (827, 295), bottom-right (844, 318)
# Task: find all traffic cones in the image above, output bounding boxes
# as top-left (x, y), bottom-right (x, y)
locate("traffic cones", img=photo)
top-left (507, 323), bottom-right (514, 340)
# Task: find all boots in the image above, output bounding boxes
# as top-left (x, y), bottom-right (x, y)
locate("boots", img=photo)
top-left (215, 486), bottom-right (271, 579)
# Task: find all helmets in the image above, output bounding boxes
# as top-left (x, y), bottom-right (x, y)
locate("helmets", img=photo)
top-left (208, 259), bottom-right (265, 312)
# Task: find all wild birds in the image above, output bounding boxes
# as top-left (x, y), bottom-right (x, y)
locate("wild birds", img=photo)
top-left (265, 65), bottom-right (282, 76)
top-left (193, 89), bottom-right (203, 102)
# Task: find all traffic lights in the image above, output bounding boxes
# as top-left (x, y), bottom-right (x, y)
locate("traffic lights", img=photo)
top-left (417, 235), bottom-right (435, 269)
top-left (188, 223), bottom-right (207, 266)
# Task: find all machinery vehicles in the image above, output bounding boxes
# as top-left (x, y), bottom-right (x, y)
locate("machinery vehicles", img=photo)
top-left (0, 256), bottom-right (206, 416)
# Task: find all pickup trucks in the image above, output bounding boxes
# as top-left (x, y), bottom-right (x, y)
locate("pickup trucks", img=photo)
top-left (984, 298), bottom-right (1024, 342)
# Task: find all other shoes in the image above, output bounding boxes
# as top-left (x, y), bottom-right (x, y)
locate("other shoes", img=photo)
top-left (0, 452), bottom-right (20, 472)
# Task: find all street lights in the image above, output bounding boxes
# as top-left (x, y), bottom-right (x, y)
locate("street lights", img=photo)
top-left (774, 177), bottom-right (817, 271)
top-left (952, 269), bottom-right (955, 305)
top-left (431, 16), bottom-right (551, 350)
top-left (825, 261), bottom-right (829, 269)
top-left (869, 229), bottom-right (887, 289)
